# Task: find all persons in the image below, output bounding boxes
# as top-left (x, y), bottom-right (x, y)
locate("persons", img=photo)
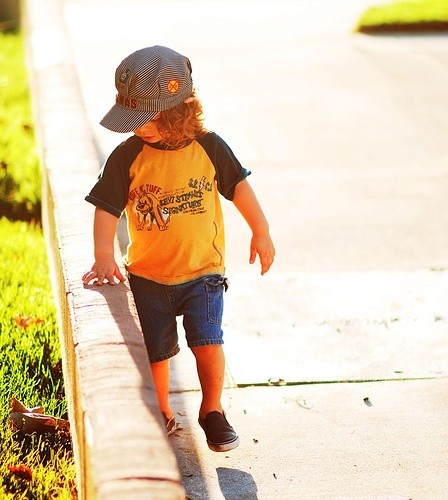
top-left (82, 45), bottom-right (276, 452)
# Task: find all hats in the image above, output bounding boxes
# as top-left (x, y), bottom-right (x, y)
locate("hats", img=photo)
top-left (99, 45), bottom-right (192, 133)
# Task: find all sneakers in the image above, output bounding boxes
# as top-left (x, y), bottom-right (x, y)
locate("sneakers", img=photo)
top-left (160, 411), bottom-right (177, 436)
top-left (198, 406), bottom-right (240, 452)
